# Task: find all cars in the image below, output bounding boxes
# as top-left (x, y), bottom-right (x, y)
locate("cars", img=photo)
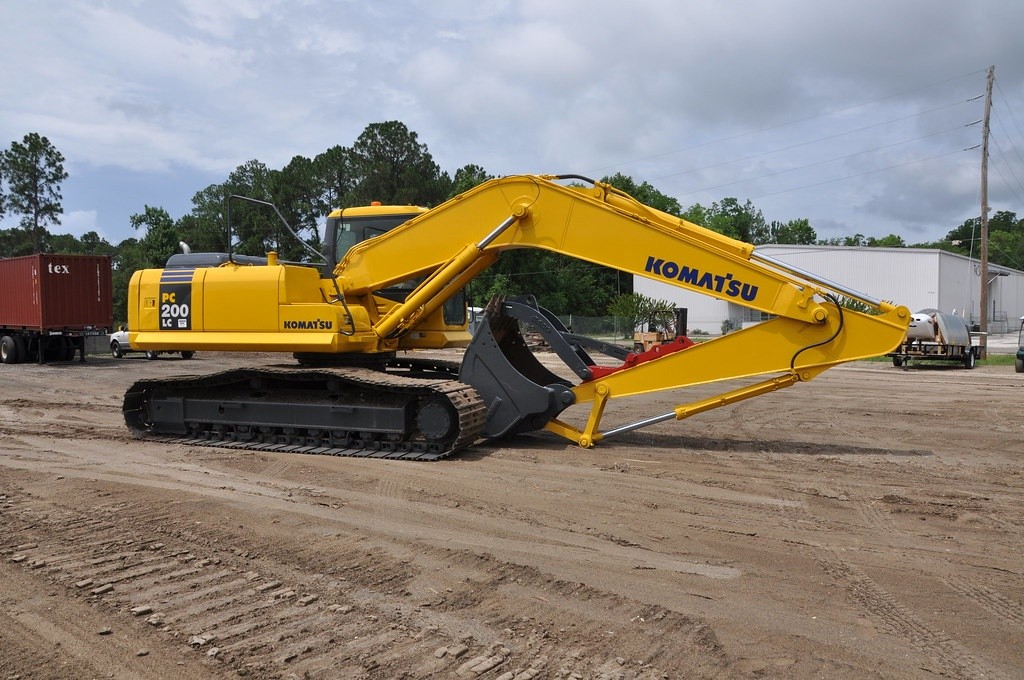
top-left (110, 320), bottom-right (195, 359)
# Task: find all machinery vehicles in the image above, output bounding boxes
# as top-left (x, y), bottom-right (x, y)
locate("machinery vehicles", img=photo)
top-left (633, 309), bottom-right (689, 353)
top-left (120, 170), bottom-right (913, 460)
